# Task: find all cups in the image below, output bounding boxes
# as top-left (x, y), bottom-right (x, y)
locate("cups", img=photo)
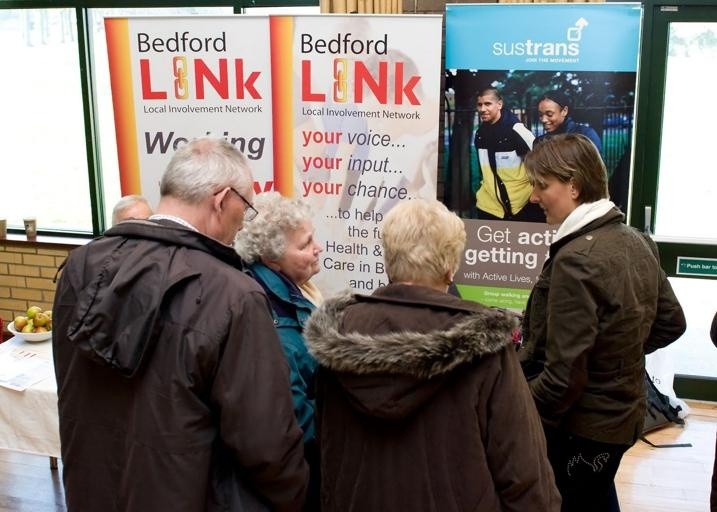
top-left (24, 219), bottom-right (36, 239)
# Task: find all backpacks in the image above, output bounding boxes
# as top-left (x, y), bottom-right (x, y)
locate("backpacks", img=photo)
top-left (639, 368), bottom-right (691, 448)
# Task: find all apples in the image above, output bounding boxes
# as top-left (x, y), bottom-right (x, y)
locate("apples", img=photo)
top-left (23, 325), bottom-right (37, 332)
top-left (28, 307), bottom-right (41, 318)
top-left (26, 318), bottom-right (34, 325)
top-left (48, 320), bottom-right (54, 328)
top-left (35, 324), bottom-right (50, 334)
top-left (15, 316), bottom-right (25, 330)
top-left (34, 313), bottom-right (49, 326)
top-left (43, 311), bottom-right (53, 317)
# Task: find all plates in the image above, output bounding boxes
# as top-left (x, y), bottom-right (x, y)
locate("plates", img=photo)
top-left (6, 322), bottom-right (51, 343)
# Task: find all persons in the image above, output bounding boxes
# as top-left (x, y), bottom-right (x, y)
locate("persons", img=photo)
top-left (232, 191), bottom-right (326, 470)
top-left (532, 91), bottom-right (604, 158)
top-left (112, 195), bottom-right (153, 223)
top-left (53, 136), bottom-right (308, 512)
top-left (473, 87), bottom-right (547, 222)
top-left (511, 133), bottom-right (686, 512)
top-left (305, 197), bottom-right (559, 511)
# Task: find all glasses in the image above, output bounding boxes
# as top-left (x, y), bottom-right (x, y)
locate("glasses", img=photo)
top-left (212, 185), bottom-right (258, 225)
